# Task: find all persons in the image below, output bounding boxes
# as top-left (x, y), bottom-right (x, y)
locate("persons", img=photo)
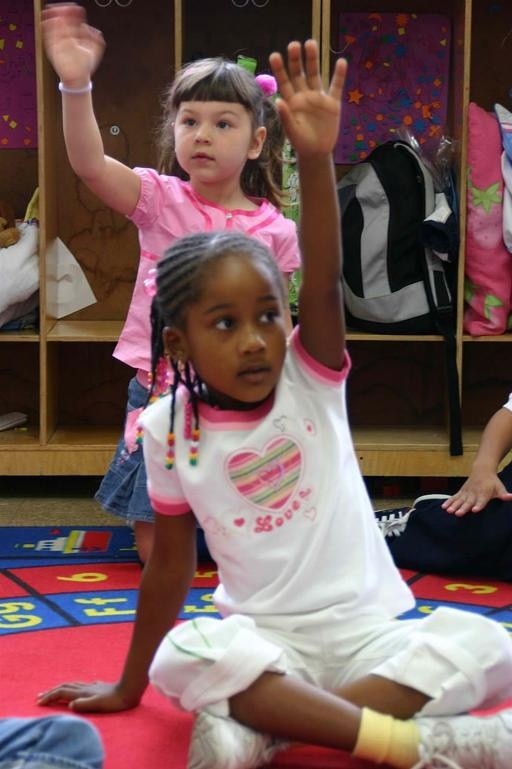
top-left (37, 37), bottom-right (512, 769)
top-left (374, 386), bottom-right (512, 581)
top-left (41, 4), bottom-right (302, 565)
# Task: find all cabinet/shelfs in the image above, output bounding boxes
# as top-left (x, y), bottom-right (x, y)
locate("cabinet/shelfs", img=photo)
top-left (1, 0), bottom-right (511, 477)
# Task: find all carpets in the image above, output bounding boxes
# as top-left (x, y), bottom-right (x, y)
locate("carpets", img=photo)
top-left (1, 523), bottom-right (511, 767)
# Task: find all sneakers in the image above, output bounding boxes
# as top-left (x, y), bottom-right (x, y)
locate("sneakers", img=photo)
top-left (187, 713), bottom-right (293, 769)
top-left (372, 494), bottom-right (453, 542)
top-left (406, 708), bottom-right (511, 769)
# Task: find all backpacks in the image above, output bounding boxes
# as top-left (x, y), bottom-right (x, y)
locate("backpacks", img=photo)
top-left (338, 139), bottom-right (452, 332)
